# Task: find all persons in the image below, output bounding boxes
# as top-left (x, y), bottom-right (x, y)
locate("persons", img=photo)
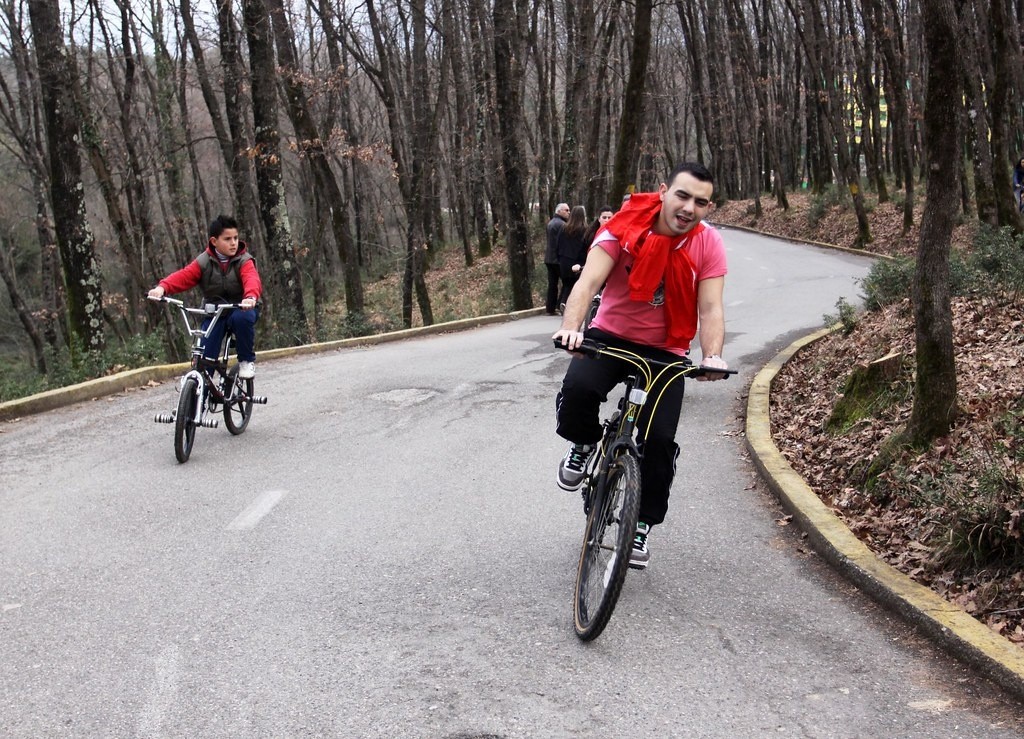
top-left (552, 163), bottom-right (727, 568)
top-left (148, 215), bottom-right (262, 417)
top-left (1012, 158), bottom-right (1024, 213)
top-left (545, 203), bottom-right (613, 315)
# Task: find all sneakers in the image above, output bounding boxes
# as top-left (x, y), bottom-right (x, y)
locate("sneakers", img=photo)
top-left (628, 521), bottom-right (650, 569)
top-left (557, 441), bottom-right (597, 491)
top-left (239, 360), bottom-right (256, 379)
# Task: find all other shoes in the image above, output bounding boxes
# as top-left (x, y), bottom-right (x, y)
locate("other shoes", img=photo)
top-left (548, 308), bottom-right (559, 315)
top-left (560, 303), bottom-right (565, 315)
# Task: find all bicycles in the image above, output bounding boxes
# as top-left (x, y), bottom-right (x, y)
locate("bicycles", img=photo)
top-left (553, 336), bottom-right (738, 642)
top-left (153, 295), bottom-right (268, 465)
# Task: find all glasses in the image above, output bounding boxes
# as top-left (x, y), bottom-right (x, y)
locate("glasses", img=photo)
top-left (564, 209), bottom-right (569, 212)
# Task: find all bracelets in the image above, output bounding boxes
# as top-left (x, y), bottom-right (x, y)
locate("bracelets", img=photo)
top-left (707, 355), bottom-right (721, 359)
top-left (247, 297), bottom-right (255, 299)
